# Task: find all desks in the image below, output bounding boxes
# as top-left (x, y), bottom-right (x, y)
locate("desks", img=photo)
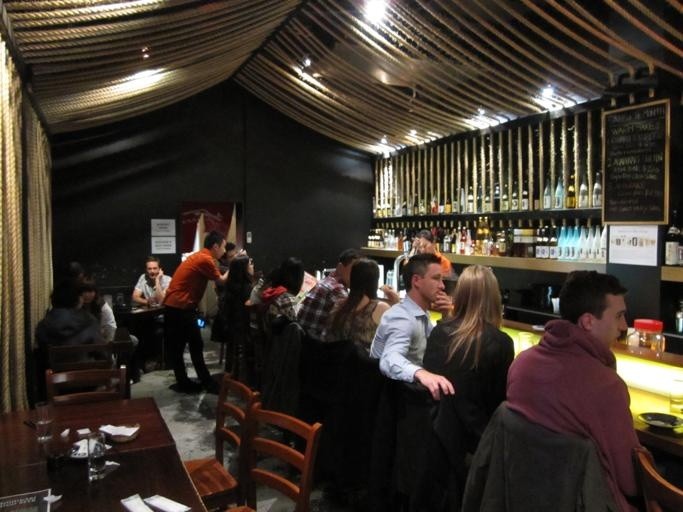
top-left (0, 445), bottom-right (211, 512)
top-left (0, 396), bottom-right (176, 469)
top-left (112, 299), bottom-right (166, 320)
top-left (108, 327), bottom-right (132, 352)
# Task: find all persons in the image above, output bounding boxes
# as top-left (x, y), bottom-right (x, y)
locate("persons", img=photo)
top-left (506, 270), bottom-right (661, 512)
top-left (35, 279), bottom-right (135, 399)
top-left (422, 265), bottom-right (513, 416)
top-left (410, 227), bottom-right (453, 277)
top-left (214, 242), bottom-right (400, 361)
top-left (74, 268), bottom-right (118, 362)
top-left (162, 231), bottom-right (249, 392)
top-left (130, 256), bottom-right (173, 310)
top-left (368, 253), bottom-right (456, 400)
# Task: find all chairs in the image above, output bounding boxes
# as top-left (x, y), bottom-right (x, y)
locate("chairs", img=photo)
top-left (180, 372), bottom-right (261, 509)
top-left (45, 362), bottom-right (126, 404)
top-left (459, 400), bottom-right (622, 511)
top-left (263, 316), bottom-right (307, 419)
top-left (46, 339), bottom-right (115, 391)
top-left (367, 378), bottom-right (466, 511)
top-left (301, 335), bottom-right (381, 481)
top-left (634, 449), bottom-right (683, 511)
top-left (232, 302), bottom-right (274, 390)
top-left (202, 404), bottom-right (323, 512)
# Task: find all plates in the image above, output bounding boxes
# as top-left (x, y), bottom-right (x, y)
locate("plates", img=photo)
top-left (637, 413), bottom-right (682, 431)
top-left (110, 424), bottom-right (140, 442)
top-left (68, 438), bottom-right (111, 458)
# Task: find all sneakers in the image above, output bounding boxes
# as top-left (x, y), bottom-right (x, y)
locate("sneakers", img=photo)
top-left (202, 380), bottom-right (221, 395)
top-left (175, 378), bottom-right (202, 394)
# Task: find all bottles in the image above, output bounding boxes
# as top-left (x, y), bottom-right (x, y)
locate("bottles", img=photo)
top-left (626, 319), bottom-right (666, 361)
top-left (662, 209), bottom-right (682, 266)
top-left (374, 172), bottom-right (602, 218)
top-left (366, 216), bottom-right (607, 258)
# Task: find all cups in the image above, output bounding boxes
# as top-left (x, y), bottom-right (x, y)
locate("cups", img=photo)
top-left (86, 432), bottom-right (107, 481)
top-left (32, 401), bottom-right (54, 442)
top-left (669, 389), bottom-right (683, 413)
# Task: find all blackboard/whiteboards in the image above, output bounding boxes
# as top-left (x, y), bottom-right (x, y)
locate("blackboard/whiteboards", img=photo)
top-left (601, 98), bottom-right (671, 225)
